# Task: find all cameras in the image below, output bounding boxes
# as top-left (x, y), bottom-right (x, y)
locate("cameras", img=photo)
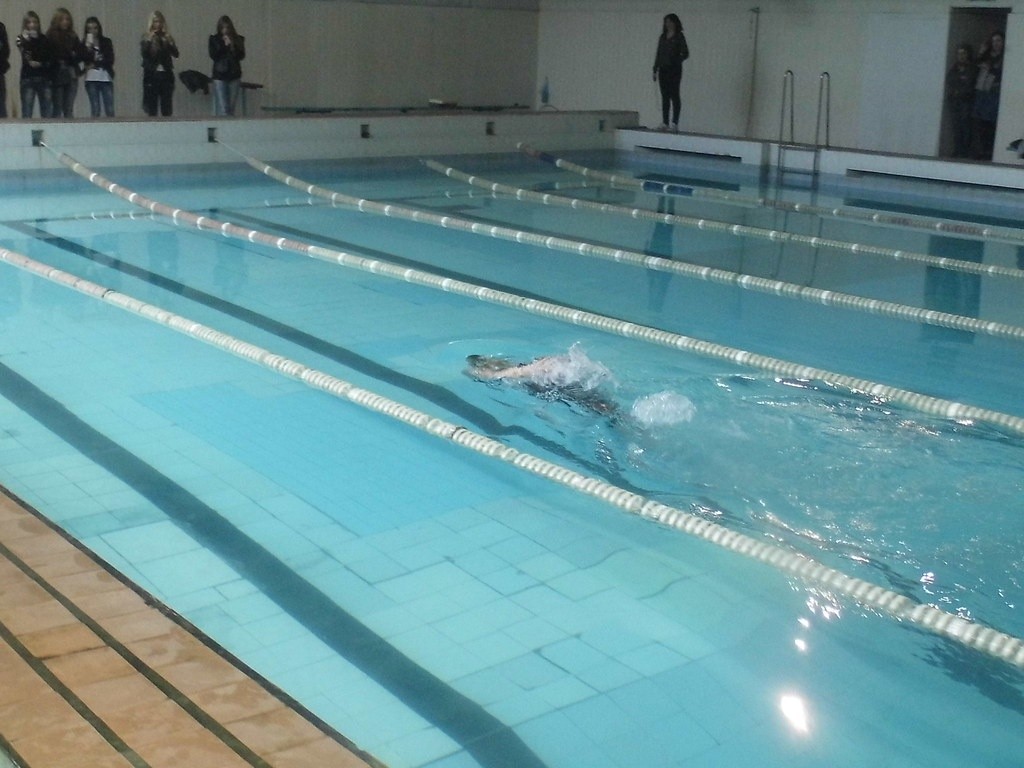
top-left (155, 29), bottom-right (164, 38)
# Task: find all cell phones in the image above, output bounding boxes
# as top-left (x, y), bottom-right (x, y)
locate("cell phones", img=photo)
top-left (87, 33), bottom-right (94, 43)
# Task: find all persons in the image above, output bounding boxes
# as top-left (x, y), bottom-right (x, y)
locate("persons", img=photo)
top-left (653, 12), bottom-right (690, 134)
top-left (0, 21), bottom-right (11, 118)
top-left (944, 34), bottom-right (1004, 160)
top-left (140, 11), bottom-right (180, 117)
top-left (45, 8), bottom-right (82, 119)
top-left (80, 17), bottom-right (115, 116)
top-left (209, 15), bottom-right (246, 115)
top-left (16, 10), bottom-right (46, 118)
